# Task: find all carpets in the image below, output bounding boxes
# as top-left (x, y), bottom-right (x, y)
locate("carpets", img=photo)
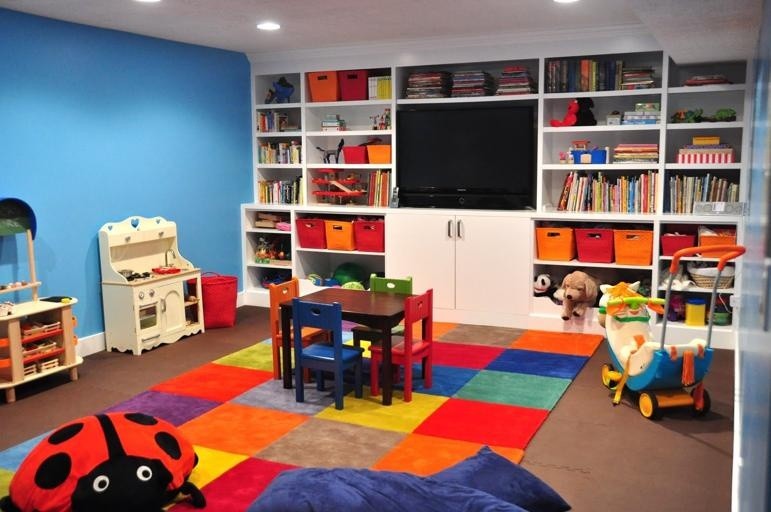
top-left (0, 319), bottom-right (609, 511)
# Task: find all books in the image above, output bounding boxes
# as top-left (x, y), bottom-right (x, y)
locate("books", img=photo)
top-left (544, 54), bottom-right (744, 217)
top-left (403, 66), bottom-right (540, 99)
top-left (254, 74), bottom-right (396, 209)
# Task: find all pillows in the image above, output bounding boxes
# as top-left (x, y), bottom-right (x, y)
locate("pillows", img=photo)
top-left (428, 445), bottom-right (574, 511)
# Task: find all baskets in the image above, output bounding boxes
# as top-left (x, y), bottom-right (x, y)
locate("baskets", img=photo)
top-left (687, 262), bottom-right (733, 288)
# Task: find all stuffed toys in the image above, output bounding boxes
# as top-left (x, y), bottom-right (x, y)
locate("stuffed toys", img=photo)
top-left (532, 270), bottom-right (566, 304)
top-left (0, 413), bottom-right (206, 512)
top-left (560, 268), bottom-right (603, 322)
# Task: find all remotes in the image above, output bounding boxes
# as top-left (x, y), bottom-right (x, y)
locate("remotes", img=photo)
top-left (392, 186), bottom-right (399, 206)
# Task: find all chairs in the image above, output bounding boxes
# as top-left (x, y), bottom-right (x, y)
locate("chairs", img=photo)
top-left (292, 297), bottom-right (365, 411)
top-left (350, 273), bottom-right (414, 384)
top-left (368, 287), bottom-right (435, 402)
top-left (265, 276), bottom-right (328, 383)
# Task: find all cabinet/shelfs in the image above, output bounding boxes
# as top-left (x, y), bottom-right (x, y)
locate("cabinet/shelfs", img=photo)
top-left (665, 54), bottom-right (754, 212)
top-left (534, 216), bottom-right (656, 339)
top-left (383, 206), bottom-right (531, 316)
top-left (0, 294), bottom-right (83, 404)
top-left (251, 62), bottom-right (395, 208)
top-left (659, 219), bottom-right (738, 331)
top-left (98, 214), bottom-right (206, 357)
top-left (536, 50), bottom-right (665, 214)
top-left (241, 203), bottom-right (293, 293)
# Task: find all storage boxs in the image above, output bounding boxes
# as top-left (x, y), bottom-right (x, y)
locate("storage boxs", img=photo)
top-left (660, 233), bottom-right (693, 258)
top-left (306, 71), bottom-right (340, 102)
top-left (613, 229), bottom-right (652, 267)
top-left (694, 226), bottom-right (737, 259)
top-left (364, 144), bottom-right (391, 164)
top-left (340, 146), bottom-right (367, 163)
top-left (571, 226), bottom-right (613, 264)
top-left (336, 68), bottom-right (367, 100)
top-left (349, 220), bottom-right (383, 252)
top-left (322, 220), bottom-right (353, 250)
top-left (294, 217), bottom-right (325, 249)
top-left (536, 227), bottom-right (576, 263)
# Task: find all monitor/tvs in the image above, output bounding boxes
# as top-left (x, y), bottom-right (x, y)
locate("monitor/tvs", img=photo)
top-left (396, 100), bottom-right (538, 208)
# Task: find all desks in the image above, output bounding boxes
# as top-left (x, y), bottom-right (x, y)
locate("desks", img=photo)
top-left (278, 286), bottom-right (424, 405)
